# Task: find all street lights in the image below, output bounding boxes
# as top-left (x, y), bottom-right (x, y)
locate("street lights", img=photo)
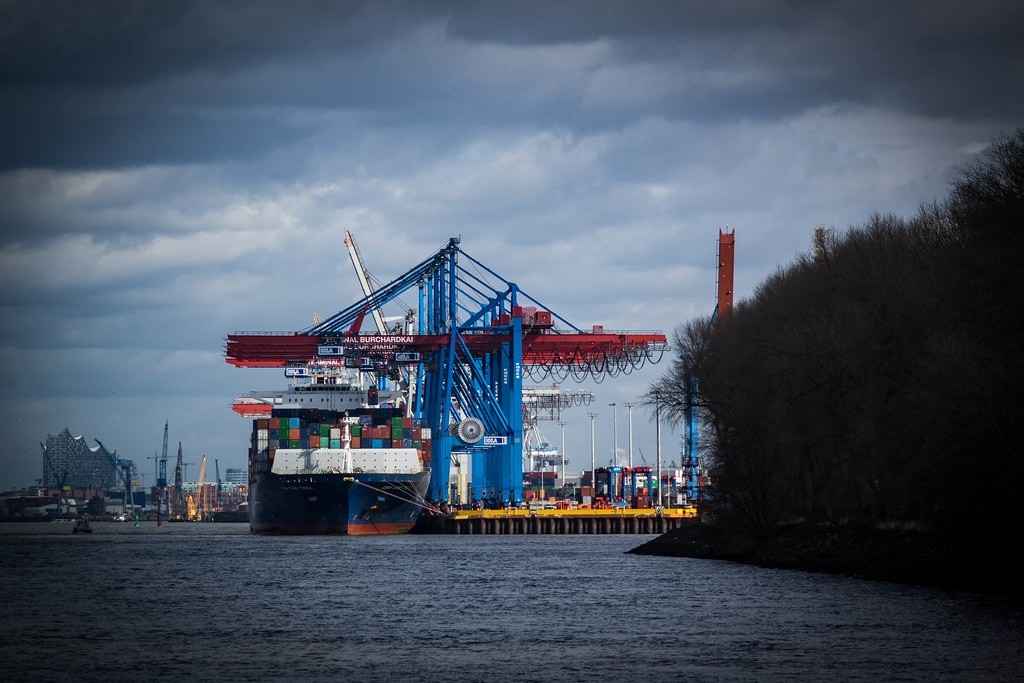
top-left (609, 402), bottom-right (619, 468)
top-left (586, 411), bottom-right (601, 486)
top-left (624, 400), bottom-right (639, 468)
top-left (558, 420), bottom-right (570, 488)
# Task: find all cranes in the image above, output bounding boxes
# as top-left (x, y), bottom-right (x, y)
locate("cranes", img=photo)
top-left (186, 450), bottom-right (210, 524)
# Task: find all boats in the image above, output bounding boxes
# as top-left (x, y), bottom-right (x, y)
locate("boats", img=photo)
top-left (246, 353), bottom-right (433, 538)
